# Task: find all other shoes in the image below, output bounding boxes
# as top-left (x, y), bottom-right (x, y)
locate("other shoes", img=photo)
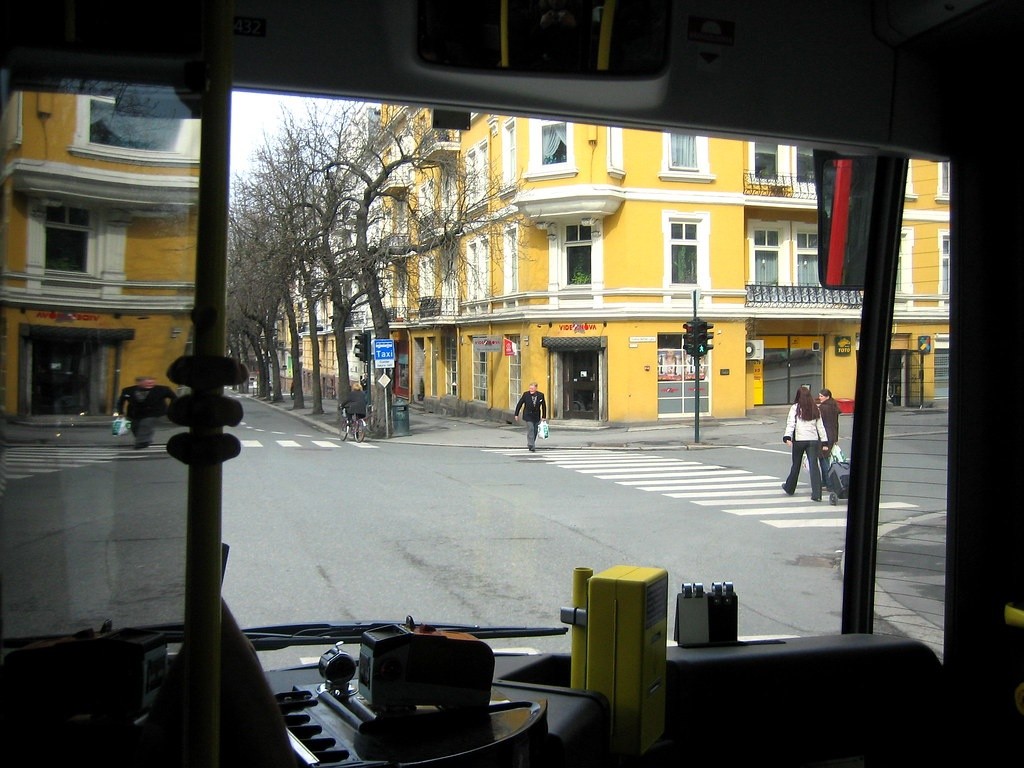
top-left (782, 482), bottom-right (793, 495)
top-left (527, 445), bottom-right (536, 451)
top-left (811, 496), bottom-right (822, 502)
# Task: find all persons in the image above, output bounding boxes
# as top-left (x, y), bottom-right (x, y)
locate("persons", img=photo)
top-left (117, 377), bottom-right (178, 450)
top-left (515, 383), bottom-right (546, 450)
top-left (782, 387), bottom-right (829, 501)
top-left (339, 373), bottom-right (369, 426)
top-left (817, 390), bottom-right (841, 486)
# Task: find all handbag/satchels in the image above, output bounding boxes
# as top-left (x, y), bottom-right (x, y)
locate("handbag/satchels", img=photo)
top-left (111, 417), bottom-right (132, 437)
top-left (802, 451), bottom-right (821, 472)
top-left (830, 444), bottom-right (846, 467)
top-left (537, 420), bottom-right (550, 438)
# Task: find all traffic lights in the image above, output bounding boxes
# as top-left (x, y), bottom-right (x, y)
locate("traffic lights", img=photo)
top-left (355, 333), bottom-right (367, 362)
top-left (696, 322), bottom-right (714, 356)
top-left (683, 322), bottom-right (694, 355)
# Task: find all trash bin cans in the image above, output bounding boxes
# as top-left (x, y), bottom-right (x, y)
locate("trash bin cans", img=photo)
top-left (392, 403), bottom-right (410, 437)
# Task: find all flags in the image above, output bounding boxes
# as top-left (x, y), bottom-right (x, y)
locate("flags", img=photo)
top-left (505, 339), bottom-right (517, 355)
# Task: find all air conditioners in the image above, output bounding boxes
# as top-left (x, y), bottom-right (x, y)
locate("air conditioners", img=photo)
top-left (745, 340), bottom-right (765, 361)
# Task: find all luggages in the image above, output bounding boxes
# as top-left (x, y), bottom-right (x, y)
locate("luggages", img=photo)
top-left (824, 449), bottom-right (851, 506)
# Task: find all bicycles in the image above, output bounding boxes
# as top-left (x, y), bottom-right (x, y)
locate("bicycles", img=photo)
top-left (338, 406), bottom-right (367, 443)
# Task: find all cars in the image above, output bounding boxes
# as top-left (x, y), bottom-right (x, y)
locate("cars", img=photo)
top-left (42, 369), bottom-right (96, 405)
